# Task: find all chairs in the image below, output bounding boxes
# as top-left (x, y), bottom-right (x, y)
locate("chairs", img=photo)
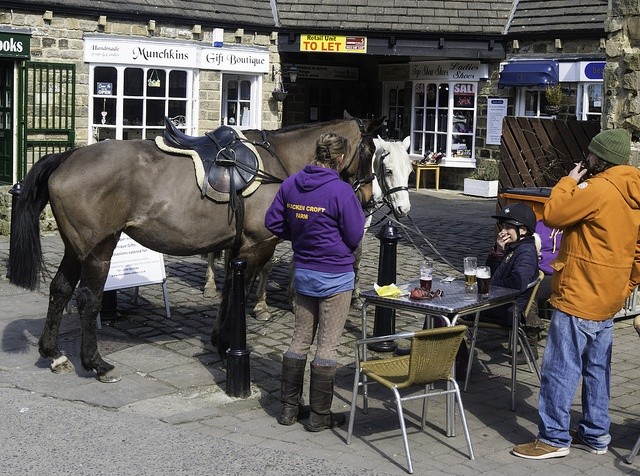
top-left (347, 325), bottom-right (474, 475)
top-left (457, 269), bottom-right (544, 394)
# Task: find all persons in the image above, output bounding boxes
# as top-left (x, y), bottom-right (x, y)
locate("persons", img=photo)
top-left (266, 133), bottom-right (365, 431)
top-left (512, 143), bottom-right (591, 462)
top-left (412, 204), bottom-right (538, 368)
top-left (510, 217), bottom-right (564, 370)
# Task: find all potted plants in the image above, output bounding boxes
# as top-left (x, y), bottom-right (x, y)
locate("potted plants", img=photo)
top-left (544, 83), bottom-right (563, 115)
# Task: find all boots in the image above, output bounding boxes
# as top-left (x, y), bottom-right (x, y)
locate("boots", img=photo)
top-left (307, 361), bottom-right (347, 432)
top-left (522, 290), bottom-right (546, 332)
top-left (281, 353), bottom-right (311, 425)
top-left (510, 332), bottom-right (538, 364)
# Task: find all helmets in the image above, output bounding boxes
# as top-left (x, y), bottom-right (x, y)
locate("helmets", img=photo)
top-left (491, 203), bottom-right (536, 241)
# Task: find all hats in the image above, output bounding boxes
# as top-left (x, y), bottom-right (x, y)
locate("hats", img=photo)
top-left (588, 128), bottom-right (632, 164)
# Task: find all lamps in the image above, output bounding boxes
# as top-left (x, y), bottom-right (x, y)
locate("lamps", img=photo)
top-left (599, 38), bottom-right (606, 52)
top-left (554, 39), bottom-right (564, 51)
top-left (513, 39), bottom-right (520, 53)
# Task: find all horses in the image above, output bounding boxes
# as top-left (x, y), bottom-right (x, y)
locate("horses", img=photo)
top-left (8, 107), bottom-right (376, 381)
top-left (199, 133), bottom-right (413, 320)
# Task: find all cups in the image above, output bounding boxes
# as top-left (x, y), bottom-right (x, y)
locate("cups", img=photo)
top-left (463, 256), bottom-right (477, 285)
top-left (475, 266), bottom-right (491, 298)
top-left (419, 260), bottom-right (434, 291)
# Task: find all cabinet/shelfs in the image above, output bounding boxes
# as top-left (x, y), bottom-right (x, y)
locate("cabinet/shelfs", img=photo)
top-left (415, 164), bottom-right (442, 192)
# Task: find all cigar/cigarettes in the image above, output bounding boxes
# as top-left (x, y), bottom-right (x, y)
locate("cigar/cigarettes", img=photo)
top-left (573, 156), bottom-right (584, 173)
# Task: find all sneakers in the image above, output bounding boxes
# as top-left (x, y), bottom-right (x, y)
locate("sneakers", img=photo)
top-left (569, 430), bottom-right (609, 454)
top-left (513, 440), bottom-right (570, 459)
